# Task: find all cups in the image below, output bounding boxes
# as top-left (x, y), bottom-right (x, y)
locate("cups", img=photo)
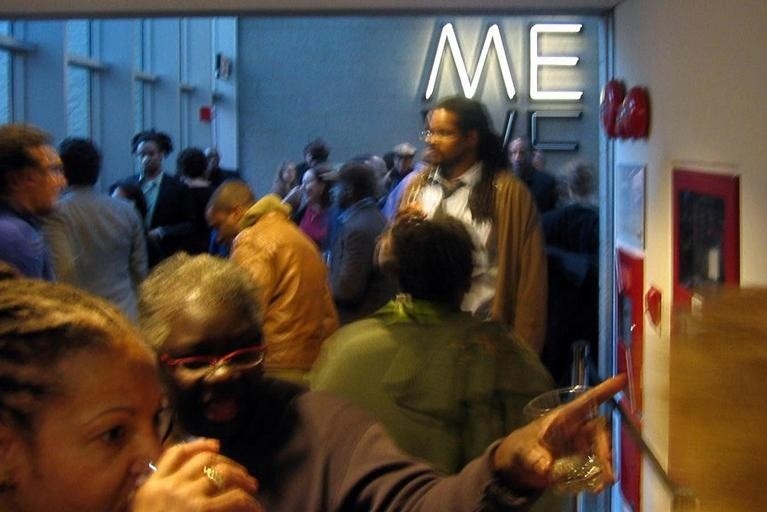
top-left (523, 386), bottom-right (608, 496)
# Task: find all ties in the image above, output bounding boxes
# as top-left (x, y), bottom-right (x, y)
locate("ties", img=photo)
top-left (434, 180), bottom-right (463, 219)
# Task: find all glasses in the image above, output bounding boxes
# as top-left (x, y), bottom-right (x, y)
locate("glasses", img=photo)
top-left (161, 337), bottom-right (268, 382)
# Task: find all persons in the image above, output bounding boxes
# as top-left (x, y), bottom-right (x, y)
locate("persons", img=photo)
top-left (372, 97), bottom-right (549, 358)
top-left (1, 124), bottom-right (628, 512)
